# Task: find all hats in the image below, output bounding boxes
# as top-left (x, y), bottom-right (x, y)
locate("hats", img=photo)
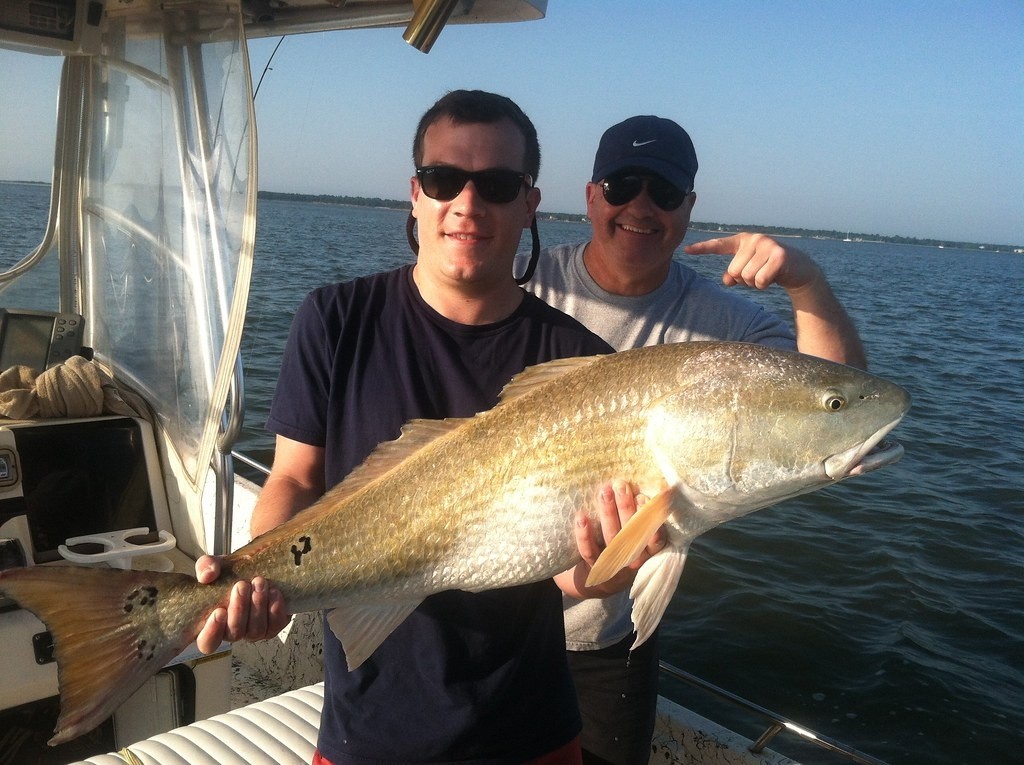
top-left (591, 115), bottom-right (698, 196)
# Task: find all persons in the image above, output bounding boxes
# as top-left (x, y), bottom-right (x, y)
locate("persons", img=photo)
top-left (194, 89), bottom-right (868, 765)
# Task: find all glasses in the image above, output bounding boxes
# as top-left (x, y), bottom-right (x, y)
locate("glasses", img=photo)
top-left (415, 166), bottom-right (532, 204)
top-left (598, 170), bottom-right (689, 211)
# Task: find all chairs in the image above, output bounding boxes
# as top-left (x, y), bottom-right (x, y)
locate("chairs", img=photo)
top-left (5, 418), bottom-right (232, 695)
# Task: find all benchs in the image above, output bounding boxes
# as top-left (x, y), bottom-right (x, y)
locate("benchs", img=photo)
top-left (60, 680), bottom-right (323, 765)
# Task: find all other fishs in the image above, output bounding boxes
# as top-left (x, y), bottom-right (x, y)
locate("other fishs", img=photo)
top-left (0, 338), bottom-right (911, 748)
